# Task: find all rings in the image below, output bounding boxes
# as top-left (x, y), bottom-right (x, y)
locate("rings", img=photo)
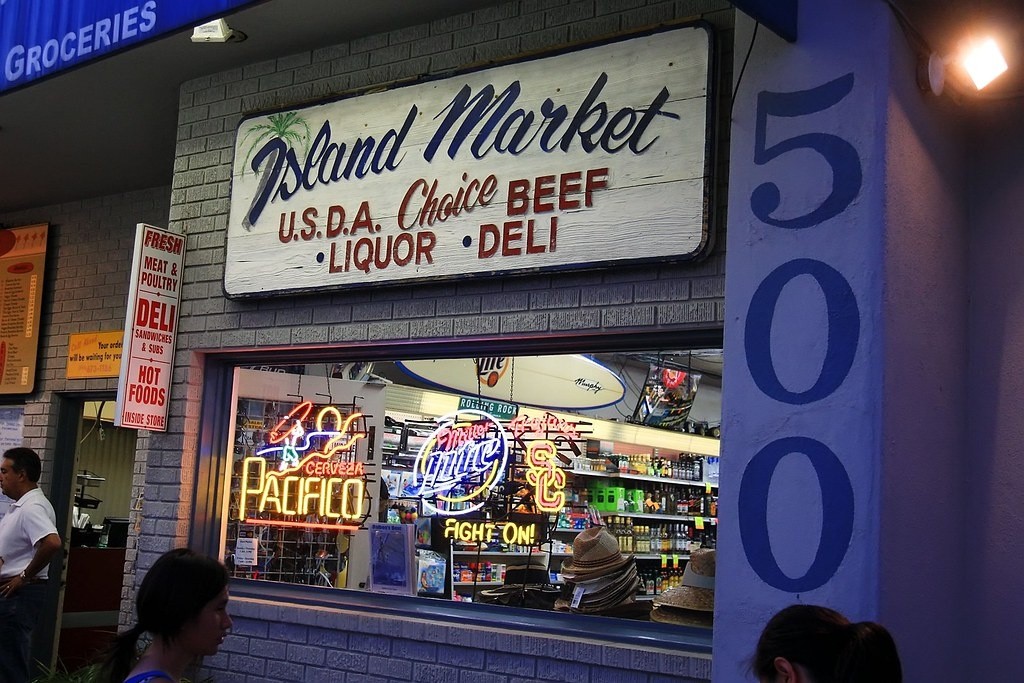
top-left (7, 585), bottom-right (10, 589)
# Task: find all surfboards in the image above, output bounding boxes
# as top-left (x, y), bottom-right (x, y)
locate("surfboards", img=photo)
top-left (393, 351), bottom-right (628, 411)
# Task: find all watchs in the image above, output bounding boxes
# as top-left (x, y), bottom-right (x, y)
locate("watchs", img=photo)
top-left (19, 570), bottom-right (32, 583)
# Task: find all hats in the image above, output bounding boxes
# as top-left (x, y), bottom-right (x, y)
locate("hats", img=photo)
top-left (650, 605), bottom-right (714, 631)
top-left (554, 526), bottom-right (641, 613)
top-left (481, 553), bottom-right (562, 595)
top-left (651, 547), bottom-right (716, 611)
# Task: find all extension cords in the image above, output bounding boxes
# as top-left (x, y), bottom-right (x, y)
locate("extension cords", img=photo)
top-left (99, 428), bottom-right (105, 441)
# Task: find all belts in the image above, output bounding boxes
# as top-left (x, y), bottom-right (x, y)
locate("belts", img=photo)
top-left (28, 578), bottom-right (48, 584)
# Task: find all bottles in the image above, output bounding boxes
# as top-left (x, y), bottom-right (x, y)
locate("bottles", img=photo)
top-left (548, 449), bottom-right (720, 596)
top-left (387, 501), bottom-right (419, 525)
top-left (452, 562), bottom-right (507, 602)
top-left (417, 525), bottom-right (428, 545)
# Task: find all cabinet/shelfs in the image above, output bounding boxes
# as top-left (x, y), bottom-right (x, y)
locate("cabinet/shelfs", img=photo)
top-left (377, 437), bottom-right (719, 602)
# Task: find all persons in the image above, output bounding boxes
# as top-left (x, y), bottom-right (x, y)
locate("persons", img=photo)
top-left (0, 447), bottom-right (61, 683)
top-left (95, 548), bottom-right (232, 683)
top-left (753, 605), bottom-right (902, 683)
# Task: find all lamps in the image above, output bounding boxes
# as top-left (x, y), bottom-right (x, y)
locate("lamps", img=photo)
top-left (189, 17), bottom-right (234, 43)
